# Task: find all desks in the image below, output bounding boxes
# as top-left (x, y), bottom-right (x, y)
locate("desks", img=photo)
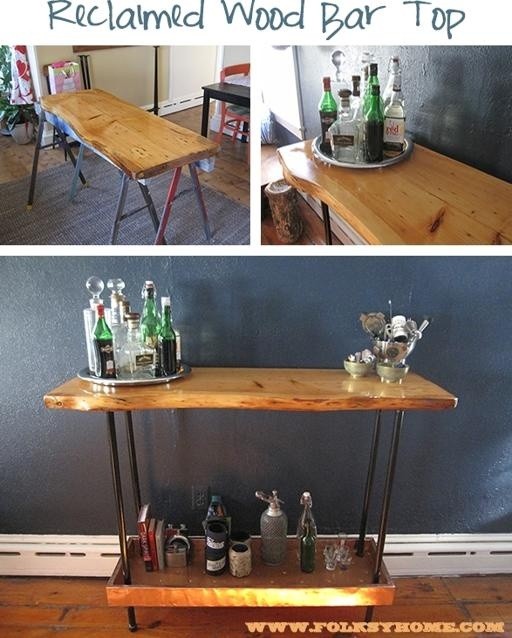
top-left (276, 140), bottom-right (512, 245)
top-left (43, 366), bottom-right (458, 632)
top-left (201, 82), bottom-right (250, 138)
top-left (26, 89), bottom-right (221, 244)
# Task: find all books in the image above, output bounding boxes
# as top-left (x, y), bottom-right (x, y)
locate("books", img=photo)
top-left (136, 503), bottom-right (166, 572)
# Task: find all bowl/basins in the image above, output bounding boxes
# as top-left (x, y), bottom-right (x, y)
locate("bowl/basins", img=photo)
top-left (343, 359), bottom-right (372, 378)
top-left (376, 363), bottom-right (410, 382)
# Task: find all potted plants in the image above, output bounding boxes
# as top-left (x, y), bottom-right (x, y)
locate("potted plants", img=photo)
top-left (0, 93), bottom-right (35, 145)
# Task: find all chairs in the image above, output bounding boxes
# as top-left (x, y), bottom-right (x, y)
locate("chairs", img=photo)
top-left (214, 63), bottom-right (251, 143)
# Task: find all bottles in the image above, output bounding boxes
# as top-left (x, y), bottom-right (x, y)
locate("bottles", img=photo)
top-left (300, 520), bottom-right (317, 573)
top-left (318, 55), bottom-right (406, 164)
top-left (93, 279), bottom-right (176, 379)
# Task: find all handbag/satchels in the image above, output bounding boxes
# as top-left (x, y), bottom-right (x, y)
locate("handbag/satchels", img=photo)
top-left (48, 61), bottom-right (80, 95)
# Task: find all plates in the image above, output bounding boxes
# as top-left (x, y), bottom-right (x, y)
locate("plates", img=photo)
top-left (310, 134), bottom-right (414, 168)
top-left (77, 361), bottom-right (191, 386)
